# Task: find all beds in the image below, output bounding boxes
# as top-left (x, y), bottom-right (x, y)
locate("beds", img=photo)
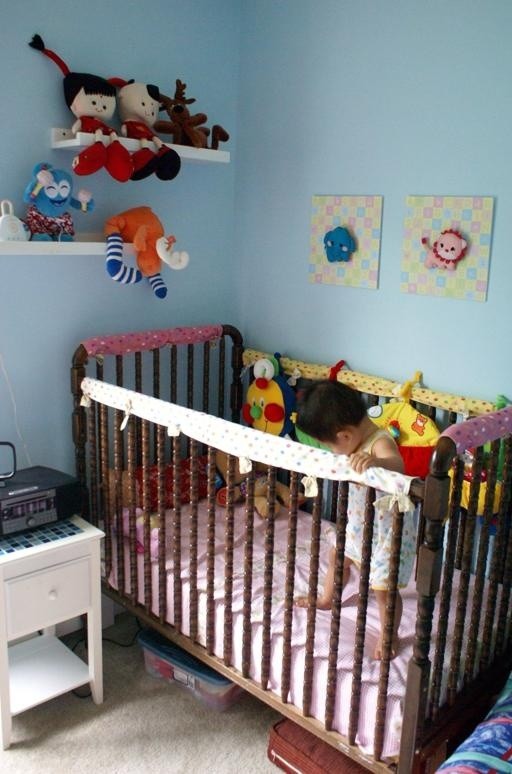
top-left (70, 323), bottom-right (510, 773)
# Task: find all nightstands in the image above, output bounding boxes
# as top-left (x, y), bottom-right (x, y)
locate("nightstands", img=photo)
top-left (1, 513), bottom-right (106, 751)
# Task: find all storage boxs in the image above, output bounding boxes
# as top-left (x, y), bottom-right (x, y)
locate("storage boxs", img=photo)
top-left (135, 627), bottom-right (249, 715)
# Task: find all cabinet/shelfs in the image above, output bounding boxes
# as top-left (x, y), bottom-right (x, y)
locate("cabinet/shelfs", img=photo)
top-left (0, 129), bottom-right (229, 255)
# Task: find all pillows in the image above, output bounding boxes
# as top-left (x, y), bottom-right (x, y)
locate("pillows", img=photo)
top-left (109, 454), bottom-right (207, 511)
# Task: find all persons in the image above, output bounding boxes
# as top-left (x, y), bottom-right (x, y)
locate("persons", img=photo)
top-left (294, 379), bottom-right (402, 661)
